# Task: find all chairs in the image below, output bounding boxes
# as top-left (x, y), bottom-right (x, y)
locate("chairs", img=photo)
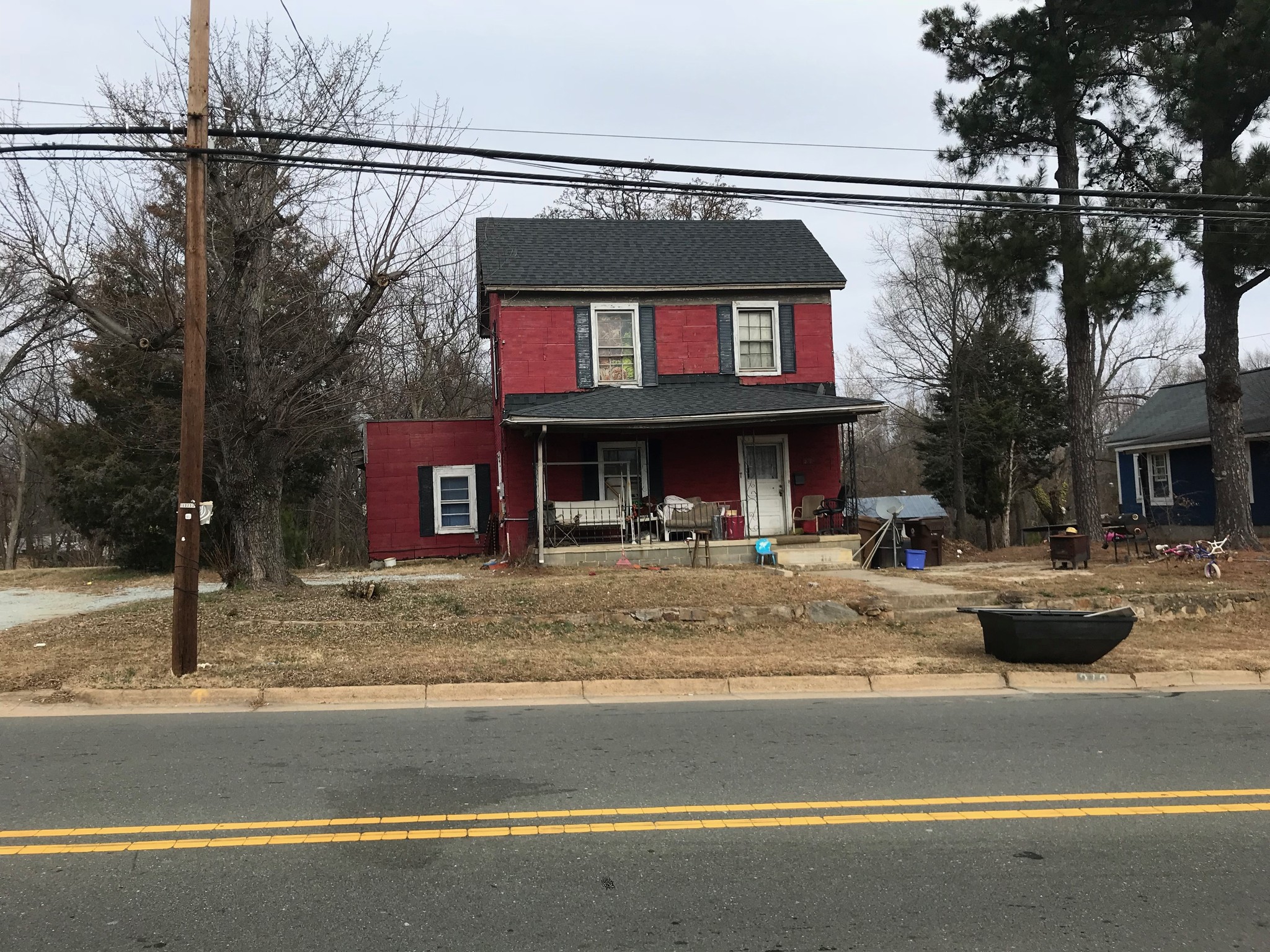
top-left (791, 495), bottom-right (825, 535)
top-left (812, 484), bottom-right (849, 535)
top-left (542, 500), bottom-right (583, 549)
top-left (755, 538), bottom-right (776, 566)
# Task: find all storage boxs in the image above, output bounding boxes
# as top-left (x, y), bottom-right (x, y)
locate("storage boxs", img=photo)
top-left (903, 549), bottom-right (927, 571)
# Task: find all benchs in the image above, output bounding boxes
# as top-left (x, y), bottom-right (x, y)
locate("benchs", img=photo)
top-left (551, 500), bottom-right (626, 530)
top-left (656, 500), bottom-right (726, 542)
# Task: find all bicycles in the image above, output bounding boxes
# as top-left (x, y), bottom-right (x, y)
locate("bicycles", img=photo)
top-left (1155, 534), bottom-right (1230, 580)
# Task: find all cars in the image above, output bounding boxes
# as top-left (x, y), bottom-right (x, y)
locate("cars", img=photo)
top-left (1104, 529), bottom-right (1126, 542)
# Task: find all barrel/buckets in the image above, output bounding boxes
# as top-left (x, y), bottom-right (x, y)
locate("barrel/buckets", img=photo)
top-left (383, 557), bottom-right (397, 567)
top-left (904, 549), bottom-right (927, 571)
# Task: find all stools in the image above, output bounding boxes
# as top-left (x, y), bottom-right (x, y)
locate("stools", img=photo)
top-left (691, 529), bottom-right (712, 569)
